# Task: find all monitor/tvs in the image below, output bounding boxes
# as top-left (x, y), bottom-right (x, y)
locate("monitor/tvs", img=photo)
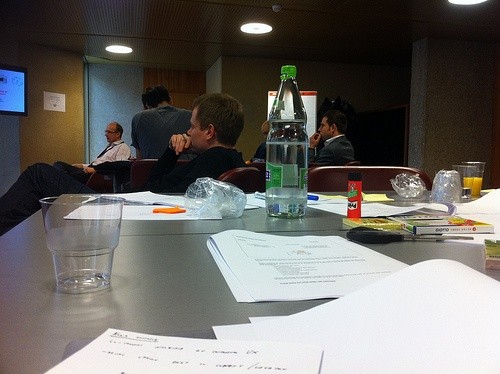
top-left (0, 63), bottom-right (29, 117)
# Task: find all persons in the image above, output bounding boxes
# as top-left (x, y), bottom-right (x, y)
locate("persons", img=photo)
top-left (0, 92), bottom-right (246, 233)
top-left (69, 122), bottom-right (132, 172)
top-left (131, 86), bottom-right (204, 158)
top-left (307, 108), bottom-right (354, 165)
top-left (246, 120), bottom-right (305, 165)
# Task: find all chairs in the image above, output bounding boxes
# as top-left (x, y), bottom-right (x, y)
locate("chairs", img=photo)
top-left (218, 167), bottom-right (263, 194)
top-left (308, 166), bottom-right (434, 192)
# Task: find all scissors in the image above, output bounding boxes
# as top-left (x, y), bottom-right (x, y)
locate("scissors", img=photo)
top-left (346, 226), bottom-right (474, 244)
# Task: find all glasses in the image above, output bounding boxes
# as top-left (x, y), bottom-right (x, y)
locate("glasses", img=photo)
top-left (105, 130), bottom-right (118, 135)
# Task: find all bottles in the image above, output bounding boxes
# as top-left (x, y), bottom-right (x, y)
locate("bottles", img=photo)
top-left (264, 65), bottom-right (308, 218)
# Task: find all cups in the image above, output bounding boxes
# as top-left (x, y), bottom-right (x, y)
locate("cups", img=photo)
top-left (451, 165), bottom-right (477, 199)
top-left (462, 161), bottom-right (486, 198)
top-left (431, 170), bottom-right (460, 204)
top-left (38, 195), bottom-right (125, 295)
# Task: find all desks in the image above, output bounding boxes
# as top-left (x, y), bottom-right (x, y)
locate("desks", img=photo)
top-left (0, 194), bottom-right (500, 374)
